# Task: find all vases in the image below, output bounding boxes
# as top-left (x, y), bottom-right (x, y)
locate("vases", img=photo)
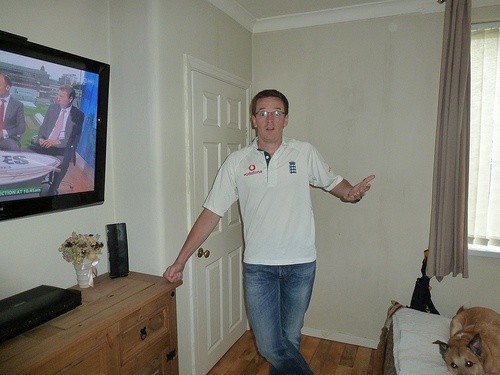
top-left (74, 264), bottom-right (95, 288)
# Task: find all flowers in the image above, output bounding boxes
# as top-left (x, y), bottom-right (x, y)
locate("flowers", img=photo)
top-left (58, 231), bottom-right (104, 267)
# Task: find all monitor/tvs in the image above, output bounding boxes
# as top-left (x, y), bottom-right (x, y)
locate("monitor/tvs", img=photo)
top-left (0, 30), bottom-right (110, 222)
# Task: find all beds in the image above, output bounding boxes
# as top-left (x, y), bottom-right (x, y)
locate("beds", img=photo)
top-left (374, 301), bottom-right (456, 375)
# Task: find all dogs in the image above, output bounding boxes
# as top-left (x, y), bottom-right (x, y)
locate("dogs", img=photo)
top-left (431, 305), bottom-right (500, 375)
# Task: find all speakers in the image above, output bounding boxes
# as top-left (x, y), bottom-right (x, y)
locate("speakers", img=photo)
top-left (105, 222), bottom-right (129, 277)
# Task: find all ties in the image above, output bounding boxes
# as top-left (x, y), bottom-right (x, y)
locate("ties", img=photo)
top-left (50, 109), bottom-right (67, 140)
top-left (0, 99), bottom-right (6, 132)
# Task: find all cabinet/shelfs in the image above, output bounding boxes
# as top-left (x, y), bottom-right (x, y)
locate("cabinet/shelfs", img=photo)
top-left (0, 267), bottom-right (183, 375)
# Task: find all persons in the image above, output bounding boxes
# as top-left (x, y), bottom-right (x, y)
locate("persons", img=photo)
top-left (163, 90), bottom-right (375, 375)
top-left (29, 86), bottom-right (85, 194)
top-left (0, 73), bottom-right (26, 151)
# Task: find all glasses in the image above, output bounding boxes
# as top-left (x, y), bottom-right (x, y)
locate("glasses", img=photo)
top-left (256, 110), bottom-right (286, 118)
